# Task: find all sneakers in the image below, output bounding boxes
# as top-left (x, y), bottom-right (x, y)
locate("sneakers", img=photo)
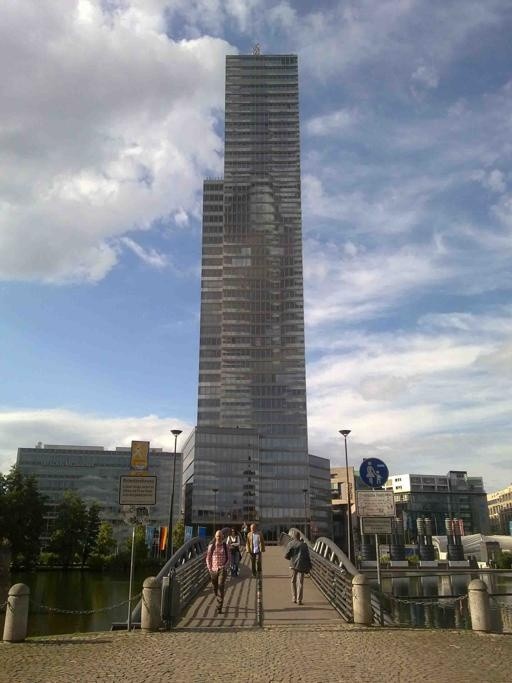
top-left (292, 597), bottom-right (304, 605)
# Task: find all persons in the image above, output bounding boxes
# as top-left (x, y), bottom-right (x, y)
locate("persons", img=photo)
top-left (205, 529), bottom-right (230, 614)
top-left (240, 520), bottom-right (249, 551)
top-left (283, 530), bottom-right (312, 605)
top-left (226, 527), bottom-right (242, 577)
top-left (245, 523), bottom-right (267, 577)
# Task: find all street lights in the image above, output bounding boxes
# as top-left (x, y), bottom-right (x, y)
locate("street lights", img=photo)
top-left (339, 430), bottom-right (355, 567)
top-left (167, 430), bottom-right (183, 557)
top-left (302, 489), bottom-right (308, 536)
top-left (212, 488), bottom-right (218, 530)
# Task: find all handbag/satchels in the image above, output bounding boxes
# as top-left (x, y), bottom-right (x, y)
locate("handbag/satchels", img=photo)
top-left (293, 549), bottom-right (307, 572)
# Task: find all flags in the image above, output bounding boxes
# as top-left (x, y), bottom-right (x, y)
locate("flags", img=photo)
top-left (145, 525), bottom-right (157, 549)
top-left (198, 526), bottom-right (206, 539)
top-left (184, 525), bottom-right (192, 543)
top-left (158, 526), bottom-right (168, 552)
